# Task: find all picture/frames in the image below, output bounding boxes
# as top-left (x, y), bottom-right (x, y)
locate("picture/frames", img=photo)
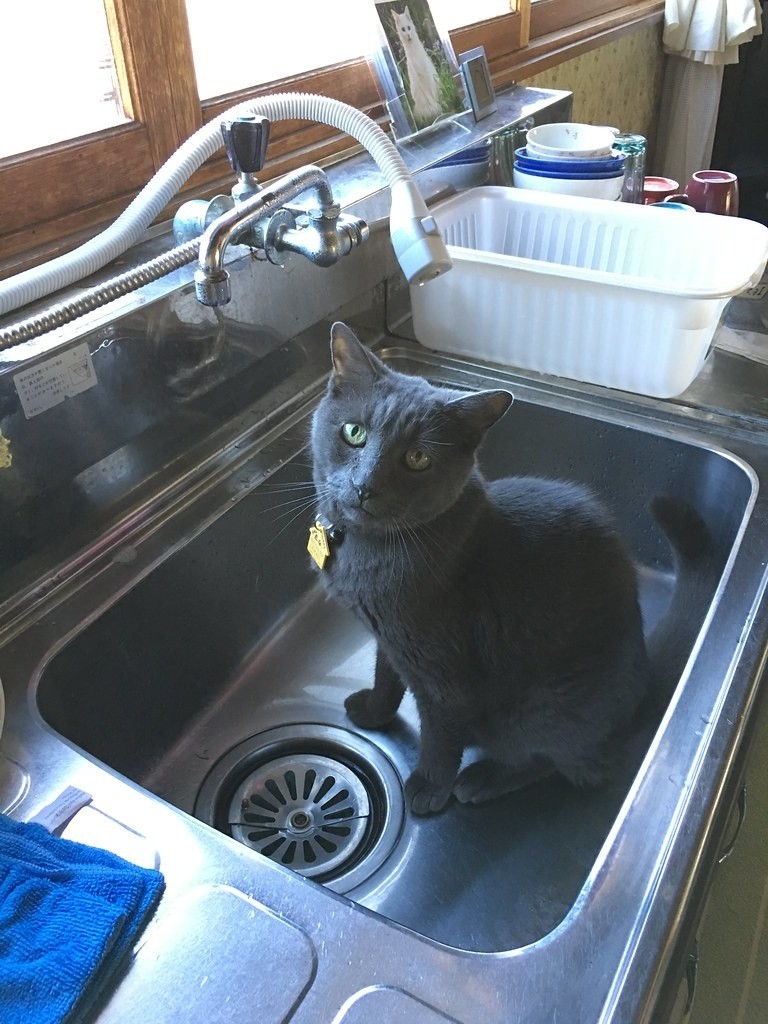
top-left (458, 45), bottom-right (498, 124)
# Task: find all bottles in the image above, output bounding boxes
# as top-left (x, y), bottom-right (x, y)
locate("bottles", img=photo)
top-left (611, 134), bottom-right (646, 204)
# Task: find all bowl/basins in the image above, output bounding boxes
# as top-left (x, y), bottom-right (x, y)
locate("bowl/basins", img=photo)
top-left (511, 122), bottom-right (625, 202)
top-left (417, 137), bottom-right (492, 193)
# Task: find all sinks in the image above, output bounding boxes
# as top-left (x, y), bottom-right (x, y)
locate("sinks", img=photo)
top-left (0, 325), bottom-right (766, 1021)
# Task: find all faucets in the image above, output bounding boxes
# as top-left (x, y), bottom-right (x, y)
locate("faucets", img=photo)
top-left (192, 162), bottom-right (344, 307)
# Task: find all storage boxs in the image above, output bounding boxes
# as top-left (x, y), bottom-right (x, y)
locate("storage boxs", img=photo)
top-left (407, 184), bottom-right (768, 400)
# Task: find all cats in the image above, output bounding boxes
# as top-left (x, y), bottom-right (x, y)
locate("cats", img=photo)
top-left (390, 3), bottom-right (446, 122)
top-left (304, 319), bottom-right (720, 818)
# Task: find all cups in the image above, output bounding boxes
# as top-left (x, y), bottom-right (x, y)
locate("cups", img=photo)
top-left (634, 170), bottom-right (739, 217)
